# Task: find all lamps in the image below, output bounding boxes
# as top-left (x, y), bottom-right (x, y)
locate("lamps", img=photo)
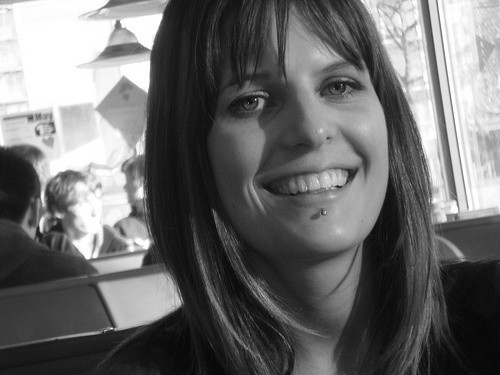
top-left (76, 18), bottom-right (153, 72)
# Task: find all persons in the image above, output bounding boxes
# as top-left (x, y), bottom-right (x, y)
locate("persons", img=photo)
top-left (111, 153), bottom-right (156, 250)
top-left (37, 169), bottom-right (138, 259)
top-left (0, 145), bottom-right (50, 185)
top-left (92, 1), bottom-right (499, 375)
top-left (0, 146), bottom-right (97, 287)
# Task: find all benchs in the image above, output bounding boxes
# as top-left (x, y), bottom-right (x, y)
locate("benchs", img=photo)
top-left (0, 201), bottom-right (500, 375)
